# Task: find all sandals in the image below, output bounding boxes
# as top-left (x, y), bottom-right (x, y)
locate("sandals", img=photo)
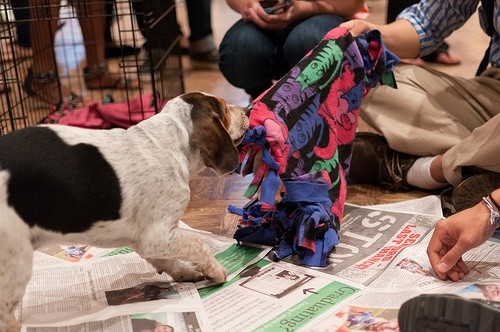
top-left (83, 61), bottom-right (144, 91)
top-left (22, 66), bottom-right (91, 110)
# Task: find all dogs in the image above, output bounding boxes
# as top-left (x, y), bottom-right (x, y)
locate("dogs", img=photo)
top-left (1, 88), bottom-right (252, 332)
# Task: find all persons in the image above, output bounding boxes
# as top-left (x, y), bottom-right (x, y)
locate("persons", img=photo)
top-left (103, 0), bottom-right (140, 58)
top-left (398, 187), bottom-right (500, 332)
top-left (386, 0), bottom-right (460, 67)
top-left (219, 0), bottom-right (370, 100)
top-left (24, 0), bottom-right (142, 109)
top-left (339, 0), bottom-right (500, 210)
top-left (132, 0), bottom-right (220, 78)
top-left (11, 0), bottom-right (33, 48)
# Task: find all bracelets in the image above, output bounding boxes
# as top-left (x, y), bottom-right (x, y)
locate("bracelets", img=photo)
top-left (489, 193), bottom-right (500, 212)
top-left (481, 197), bottom-right (500, 225)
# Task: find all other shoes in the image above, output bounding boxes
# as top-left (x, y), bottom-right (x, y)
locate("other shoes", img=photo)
top-left (452, 170), bottom-right (500, 232)
top-left (137, 49), bottom-right (169, 80)
top-left (398, 294), bottom-right (500, 332)
top-left (105, 44), bottom-right (141, 60)
top-left (188, 48), bottom-right (221, 72)
top-left (435, 50), bottom-right (459, 65)
top-left (349, 132), bottom-right (454, 193)
